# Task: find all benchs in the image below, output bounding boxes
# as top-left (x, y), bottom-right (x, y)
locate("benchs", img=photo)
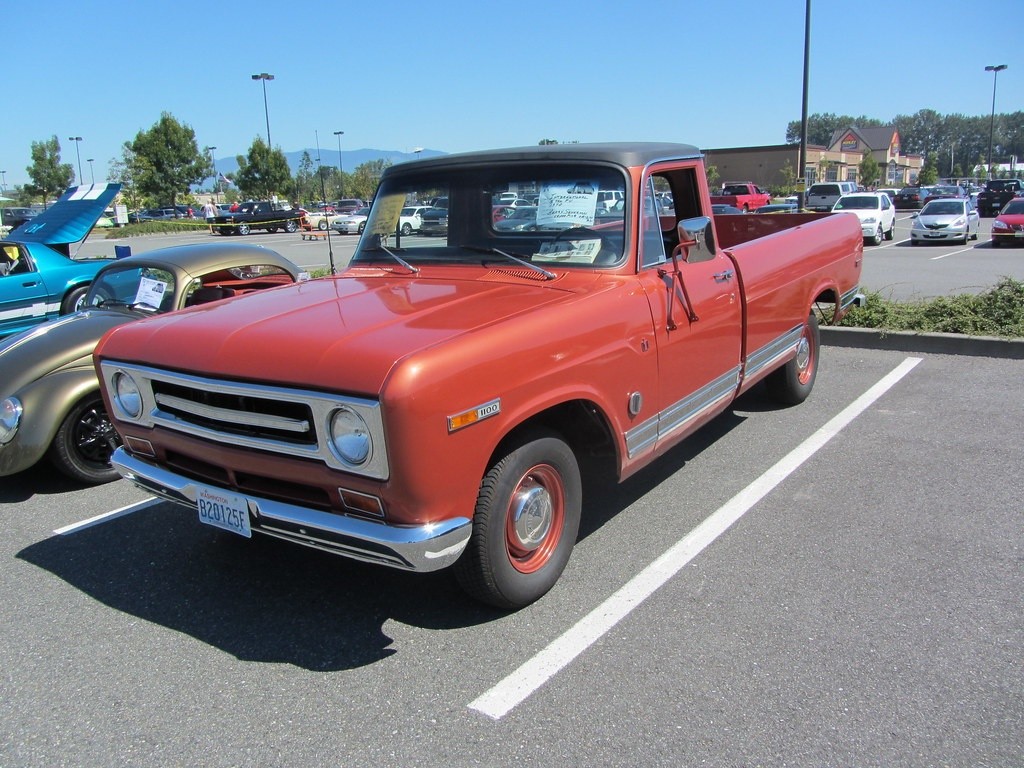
top-left (491, 237), bottom-right (672, 265)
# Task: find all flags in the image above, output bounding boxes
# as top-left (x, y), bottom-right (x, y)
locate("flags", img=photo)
top-left (220, 173), bottom-right (230, 182)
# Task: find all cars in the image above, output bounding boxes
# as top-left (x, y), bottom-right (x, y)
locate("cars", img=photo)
top-left (874, 177), bottom-right (1024, 217)
top-left (330, 206), bottom-right (373, 236)
top-left (829, 189), bottom-right (897, 246)
top-left (1, 207), bottom-right (40, 227)
top-left (805, 181), bottom-right (859, 211)
top-left (711, 204), bottom-right (744, 214)
top-left (0, 180), bottom-right (124, 346)
top-left (418, 189), bottom-right (675, 239)
top-left (990, 198), bottom-right (1024, 249)
top-left (391, 205), bottom-right (433, 237)
top-left (0, 242), bottom-right (313, 489)
top-left (754, 203), bottom-right (798, 216)
top-left (910, 198), bottom-right (981, 245)
top-left (96, 203), bottom-right (236, 229)
top-left (271, 198), bottom-right (366, 231)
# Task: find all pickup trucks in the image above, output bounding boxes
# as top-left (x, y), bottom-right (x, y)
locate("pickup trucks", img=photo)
top-left (709, 181), bottom-right (773, 213)
top-left (206, 200), bottom-right (309, 236)
top-left (92, 140), bottom-right (865, 608)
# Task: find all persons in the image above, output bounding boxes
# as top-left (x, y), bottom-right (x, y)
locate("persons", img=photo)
top-left (188, 207), bottom-right (193, 217)
top-left (203, 199), bottom-right (218, 234)
top-left (230, 202), bottom-right (239, 211)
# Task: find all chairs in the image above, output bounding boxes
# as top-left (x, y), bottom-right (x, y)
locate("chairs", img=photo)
top-left (115, 245), bottom-right (131, 258)
top-left (190, 288), bottom-right (235, 305)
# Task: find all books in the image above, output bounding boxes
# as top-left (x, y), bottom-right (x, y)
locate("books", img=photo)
top-left (532, 239), bottom-right (602, 264)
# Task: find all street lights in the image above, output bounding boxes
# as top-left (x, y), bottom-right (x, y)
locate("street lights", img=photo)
top-left (0, 170), bottom-right (8, 192)
top-left (314, 157), bottom-right (320, 174)
top-left (985, 64), bottom-right (1009, 172)
top-left (68, 135), bottom-right (84, 187)
top-left (208, 146), bottom-right (220, 205)
top-left (86, 158), bottom-right (94, 182)
top-left (332, 132), bottom-right (345, 200)
